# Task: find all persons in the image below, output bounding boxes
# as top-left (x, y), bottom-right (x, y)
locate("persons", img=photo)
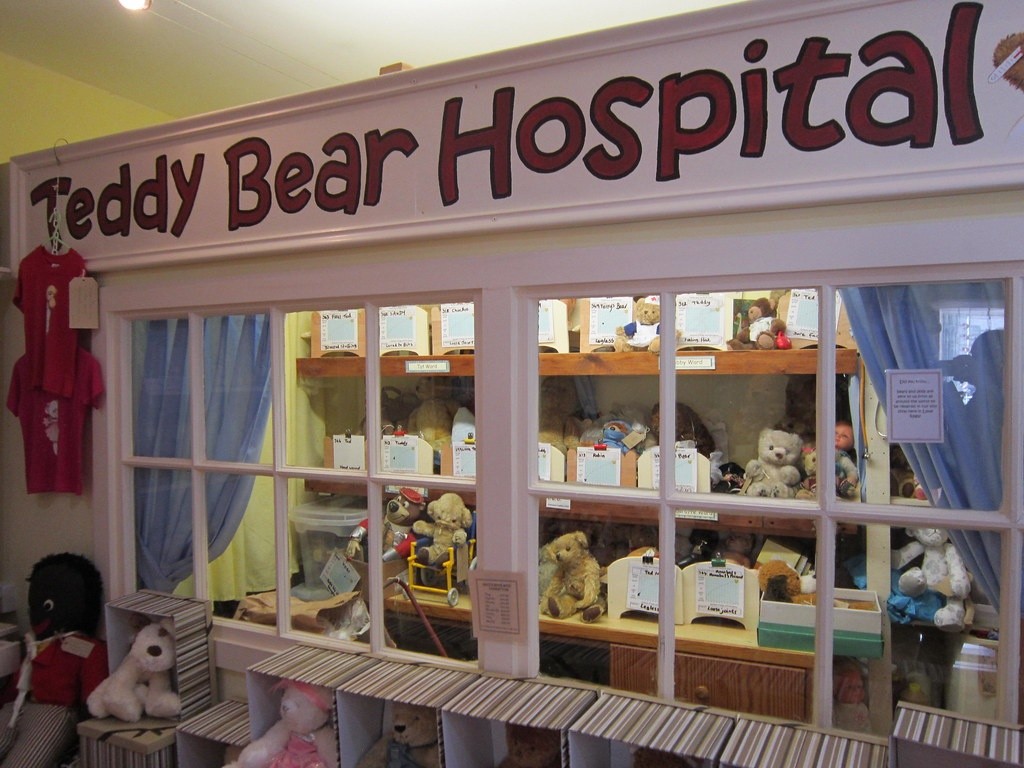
top-left (835, 421), bottom-right (859, 495)
top-left (834, 666), bottom-right (872, 736)
top-left (718, 530), bottom-right (754, 568)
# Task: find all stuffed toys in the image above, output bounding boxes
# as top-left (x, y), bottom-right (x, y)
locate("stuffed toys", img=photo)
top-left (219, 679), bottom-right (339, 768)
top-left (493, 723), bottom-right (562, 768)
top-left (913, 476), bottom-right (927, 499)
top-left (346, 488), bottom-right (424, 562)
top-left (795, 446), bottom-right (817, 501)
top-left (775, 378), bottom-right (816, 442)
top-left (889, 448), bottom-right (915, 498)
top-left (412, 494), bottom-right (472, 568)
top-left (759, 560), bottom-right (878, 612)
top-left (87, 614), bottom-right (180, 723)
top-left (354, 702), bottom-right (440, 767)
top-left (407, 376), bottom-right (460, 452)
top-left (614, 296), bottom-right (682, 352)
top-left (598, 419), bottom-right (633, 454)
top-left (540, 531), bottom-right (607, 623)
top-left (742, 427), bottom-right (803, 499)
top-left (890, 527), bottom-right (972, 633)
top-left (633, 749), bottom-right (704, 768)
top-left (540, 376), bottom-right (582, 458)
top-left (0, 553), bottom-right (108, 768)
top-left (726, 297), bottom-right (786, 350)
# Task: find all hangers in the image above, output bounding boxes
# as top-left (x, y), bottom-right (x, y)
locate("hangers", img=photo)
top-left (44, 210), bottom-right (71, 255)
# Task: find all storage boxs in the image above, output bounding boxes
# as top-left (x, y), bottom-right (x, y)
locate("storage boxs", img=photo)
top-left (290, 287), bottom-right (884, 659)
top-left (1, 588), bottom-right (1023, 767)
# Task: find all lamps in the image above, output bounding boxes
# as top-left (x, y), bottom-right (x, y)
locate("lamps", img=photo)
top-left (119, 0), bottom-right (152, 12)
